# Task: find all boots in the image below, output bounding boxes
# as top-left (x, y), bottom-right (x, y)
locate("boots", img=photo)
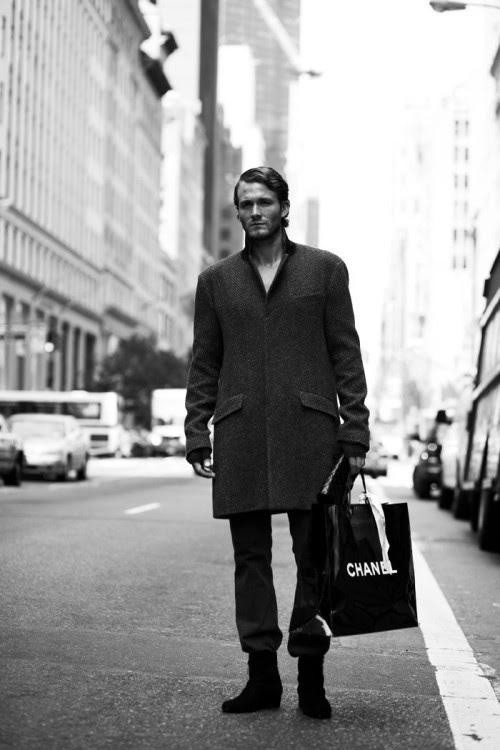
top-left (298, 655), bottom-right (331, 719)
top-left (222, 652), bottom-right (283, 713)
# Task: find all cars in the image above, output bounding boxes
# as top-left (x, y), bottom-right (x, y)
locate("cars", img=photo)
top-left (412, 246), bottom-right (500, 554)
top-left (0, 388), bottom-right (215, 486)
top-left (359, 441), bottom-right (388, 479)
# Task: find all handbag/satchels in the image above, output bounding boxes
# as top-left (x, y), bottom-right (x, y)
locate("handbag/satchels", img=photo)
top-left (316, 503), bottom-right (418, 637)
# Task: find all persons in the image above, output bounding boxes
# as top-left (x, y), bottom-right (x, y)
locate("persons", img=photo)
top-left (181, 166), bottom-right (372, 721)
